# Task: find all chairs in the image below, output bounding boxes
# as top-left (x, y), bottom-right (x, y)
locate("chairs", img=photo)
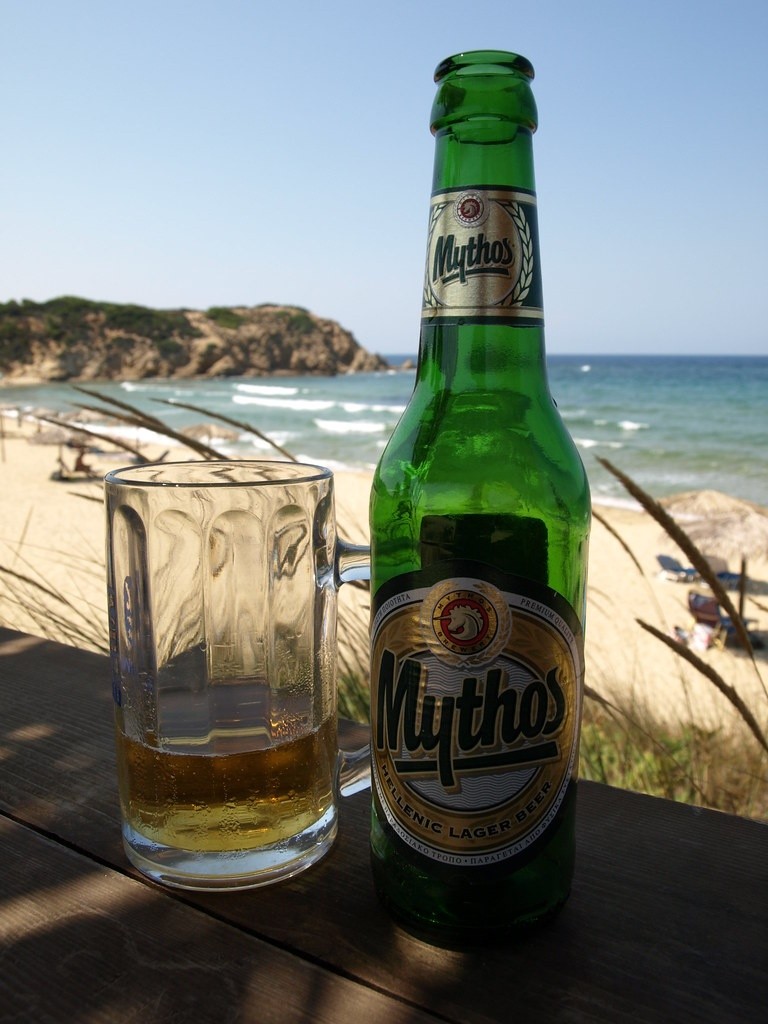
top-left (57, 457), bottom-right (104, 480)
top-left (657, 554), bottom-right (701, 582)
top-left (688, 591), bottom-right (762, 647)
top-left (703, 556), bottom-right (747, 588)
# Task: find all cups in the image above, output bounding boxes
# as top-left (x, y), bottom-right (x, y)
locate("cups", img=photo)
top-left (105, 460), bottom-right (372, 892)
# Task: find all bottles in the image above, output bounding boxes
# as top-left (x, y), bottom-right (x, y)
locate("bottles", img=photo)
top-left (371, 50), bottom-right (591, 952)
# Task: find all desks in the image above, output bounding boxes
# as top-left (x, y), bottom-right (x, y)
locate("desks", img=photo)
top-left (0, 627), bottom-right (768, 1024)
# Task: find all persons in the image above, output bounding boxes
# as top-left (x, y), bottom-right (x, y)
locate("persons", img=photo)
top-left (73, 451), bottom-right (90, 472)
